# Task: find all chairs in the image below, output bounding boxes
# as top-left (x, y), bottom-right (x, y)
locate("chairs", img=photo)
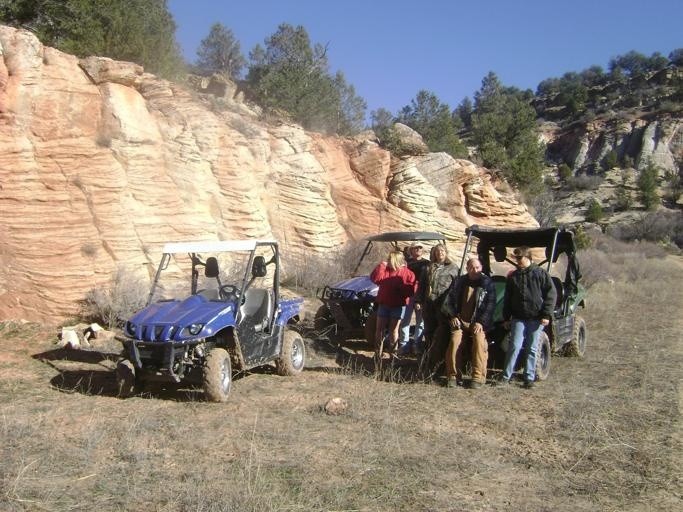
top-left (196, 287), bottom-right (271, 332)
top-left (492, 273), bottom-right (566, 317)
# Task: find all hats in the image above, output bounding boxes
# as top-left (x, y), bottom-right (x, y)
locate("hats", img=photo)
top-left (409, 243), bottom-right (422, 247)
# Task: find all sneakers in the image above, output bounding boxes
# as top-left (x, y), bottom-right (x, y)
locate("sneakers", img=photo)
top-left (526, 380), bottom-right (535, 389)
top-left (497, 378), bottom-right (510, 386)
top-left (446, 379), bottom-right (458, 389)
top-left (469, 381), bottom-right (485, 390)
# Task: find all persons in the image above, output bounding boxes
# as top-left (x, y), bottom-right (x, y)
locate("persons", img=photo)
top-left (440, 258), bottom-right (496, 389)
top-left (397, 241), bottom-right (433, 359)
top-left (492, 244), bottom-right (559, 390)
top-left (370, 250), bottom-right (419, 370)
top-left (413, 242), bottom-right (463, 382)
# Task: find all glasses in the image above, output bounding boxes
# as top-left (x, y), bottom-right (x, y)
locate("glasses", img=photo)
top-left (515, 255), bottom-right (526, 259)
